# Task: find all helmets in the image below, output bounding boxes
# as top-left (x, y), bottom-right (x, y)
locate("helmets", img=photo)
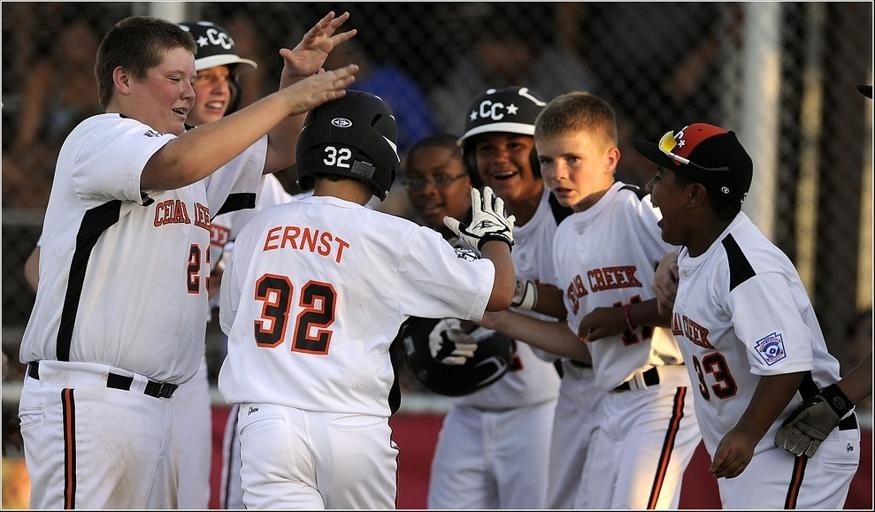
top-left (175, 20), bottom-right (258, 117)
top-left (453, 85), bottom-right (549, 190)
top-left (400, 316), bottom-right (517, 397)
top-left (295, 88), bottom-right (400, 203)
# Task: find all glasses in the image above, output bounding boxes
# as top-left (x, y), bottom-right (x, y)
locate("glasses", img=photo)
top-left (400, 168), bottom-right (468, 192)
top-left (659, 129), bottom-right (730, 174)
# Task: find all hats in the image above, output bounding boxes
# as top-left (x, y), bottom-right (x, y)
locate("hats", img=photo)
top-left (631, 122), bottom-right (753, 205)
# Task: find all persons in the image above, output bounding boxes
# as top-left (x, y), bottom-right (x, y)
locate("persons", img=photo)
top-left (577, 124), bottom-right (861, 512)
top-left (17, 10), bottom-right (359, 512)
top-left (220, 92), bottom-right (516, 512)
top-left (24, 20), bottom-right (269, 293)
top-left (403, 132), bottom-right (473, 239)
top-left (477, 90), bottom-right (703, 512)
top-left (344, 26), bottom-right (726, 187)
top-left (778, 352), bottom-right (874, 457)
top-left (427, 88), bottom-right (564, 512)
top-left (2, 15), bottom-right (100, 217)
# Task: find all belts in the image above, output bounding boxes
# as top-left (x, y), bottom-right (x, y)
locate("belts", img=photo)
top-left (615, 365), bottom-right (659, 391)
top-left (27, 360), bottom-right (178, 400)
top-left (838, 411), bottom-right (859, 431)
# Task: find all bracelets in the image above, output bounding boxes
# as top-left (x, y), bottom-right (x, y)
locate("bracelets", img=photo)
top-left (624, 304), bottom-right (634, 331)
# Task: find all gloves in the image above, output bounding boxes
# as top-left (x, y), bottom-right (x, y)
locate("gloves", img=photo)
top-left (442, 184), bottom-right (517, 253)
top-left (774, 383), bottom-right (854, 459)
